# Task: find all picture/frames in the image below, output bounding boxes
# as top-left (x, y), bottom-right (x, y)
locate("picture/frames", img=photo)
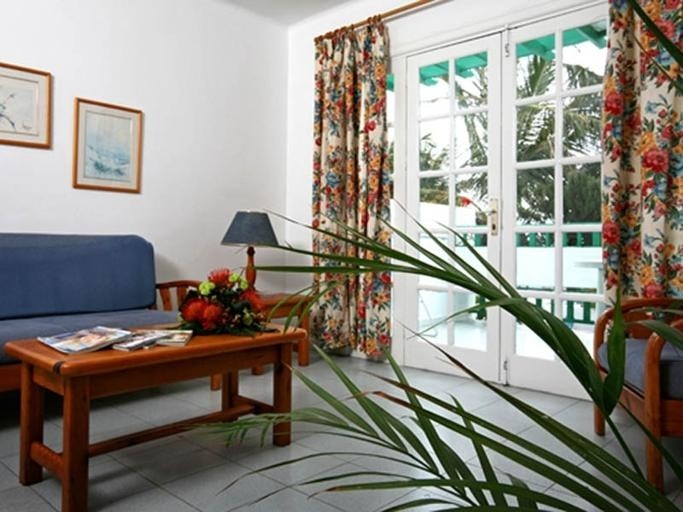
top-left (0, 63), bottom-right (53, 149)
top-left (74, 97), bottom-right (143, 194)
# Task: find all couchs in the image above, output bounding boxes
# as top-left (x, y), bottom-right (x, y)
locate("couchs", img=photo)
top-left (592, 296), bottom-right (683, 494)
top-left (0, 233), bottom-right (181, 394)
top-left (153, 279), bottom-right (224, 390)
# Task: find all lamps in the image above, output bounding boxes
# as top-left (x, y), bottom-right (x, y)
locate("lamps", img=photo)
top-left (221, 211), bottom-right (278, 293)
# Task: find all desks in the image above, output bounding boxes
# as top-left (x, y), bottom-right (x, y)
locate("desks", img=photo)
top-left (252, 293), bottom-right (313, 375)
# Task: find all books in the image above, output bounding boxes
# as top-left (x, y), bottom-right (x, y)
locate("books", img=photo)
top-left (154, 330), bottom-right (193, 348)
top-left (110, 328), bottom-right (173, 351)
top-left (37, 326), bottom-right (131, 355)
